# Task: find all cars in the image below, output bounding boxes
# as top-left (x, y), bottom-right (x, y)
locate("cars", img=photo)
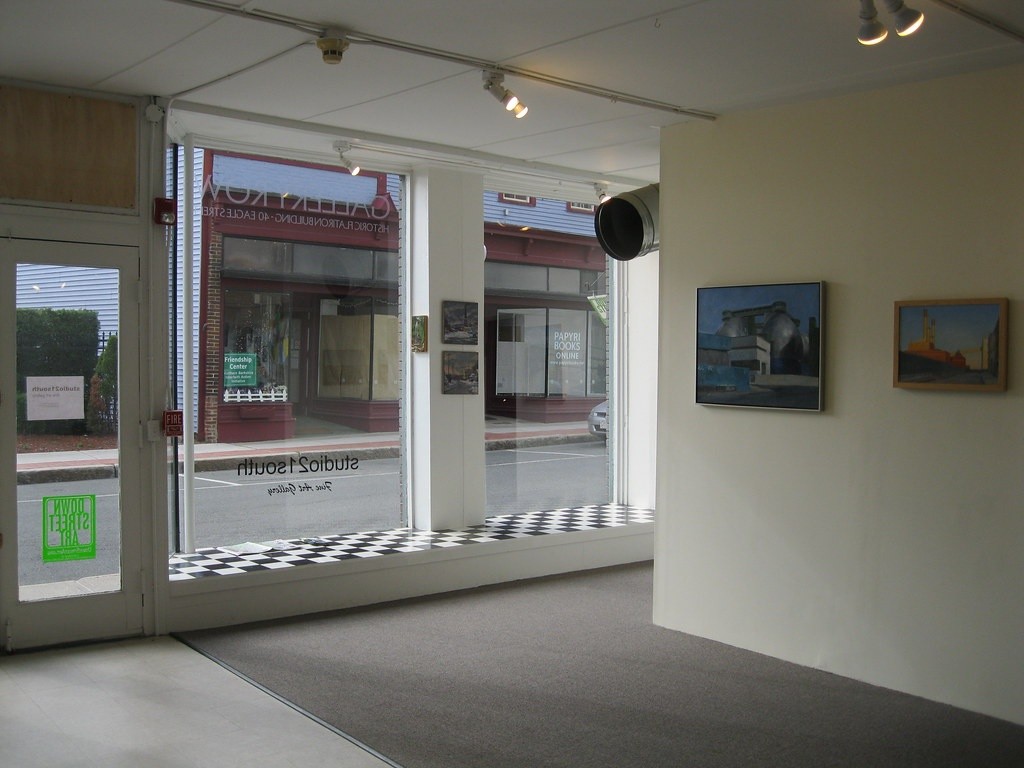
top-left (585, 399), bottom-right (608, 444)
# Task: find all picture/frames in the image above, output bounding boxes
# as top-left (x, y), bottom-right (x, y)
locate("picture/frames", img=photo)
top-left (695, 279), bottom-right (826, 412)
top-left (892, 297), bottom-right (1009, 392)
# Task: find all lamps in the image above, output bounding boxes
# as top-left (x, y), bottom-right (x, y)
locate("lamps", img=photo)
top-left (481, 69), bottom-right (528, 119)
top-left (594, 182), bottom-right (611, 204)
top-left (856, 0), bottom-right (888, 46)
top-left (331, 140), bottom-right (360, 177)
top-left (886, 0), bottom-right (924, 37)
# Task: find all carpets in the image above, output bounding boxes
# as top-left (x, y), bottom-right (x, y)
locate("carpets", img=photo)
top-left (170, 557), bottom-right (1024, 768)
